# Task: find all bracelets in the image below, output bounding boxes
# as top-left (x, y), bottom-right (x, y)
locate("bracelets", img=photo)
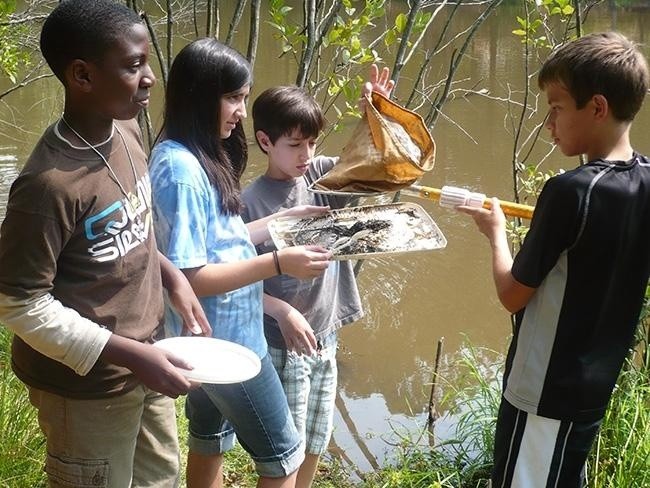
top-left (271, 250), bottom-right (282, 276)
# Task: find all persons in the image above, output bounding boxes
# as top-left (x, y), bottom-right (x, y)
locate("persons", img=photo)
top-left (0, 0), bottom-right (212, 488)
top-left (150, 36), bottom-right (330, 488)
top-left (235, 64), bottom-right (395, 487)
top-left (452, 33), bottom-right (648, 487)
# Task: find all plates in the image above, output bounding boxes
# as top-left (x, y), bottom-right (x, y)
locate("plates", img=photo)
top-left (150, 336), bottom-right (262, 385)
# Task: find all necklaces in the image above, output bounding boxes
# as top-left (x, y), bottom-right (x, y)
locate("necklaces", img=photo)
top-left (60, 112), bottom-right (142, 227)
